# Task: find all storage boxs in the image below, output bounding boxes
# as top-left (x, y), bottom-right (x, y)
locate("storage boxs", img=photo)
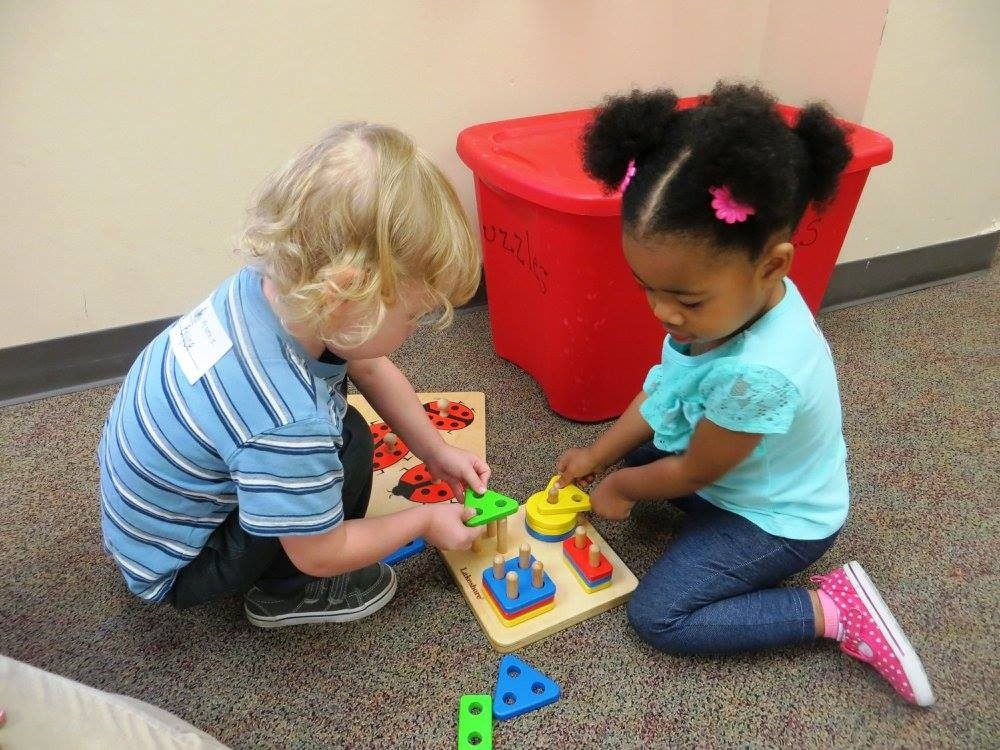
top-left (454, 83), bottom-right (893, 422)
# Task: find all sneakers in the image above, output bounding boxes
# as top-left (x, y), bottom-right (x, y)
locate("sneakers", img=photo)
top-left (809, 560), bottom-right (935, 708)
top-left (242, 560), bottom-right (399, 630)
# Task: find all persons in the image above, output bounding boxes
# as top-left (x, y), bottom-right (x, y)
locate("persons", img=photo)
top-left (101, 120), bottom-right (491, 627)
top-left (553, 81), bottom-right (934, 708)
